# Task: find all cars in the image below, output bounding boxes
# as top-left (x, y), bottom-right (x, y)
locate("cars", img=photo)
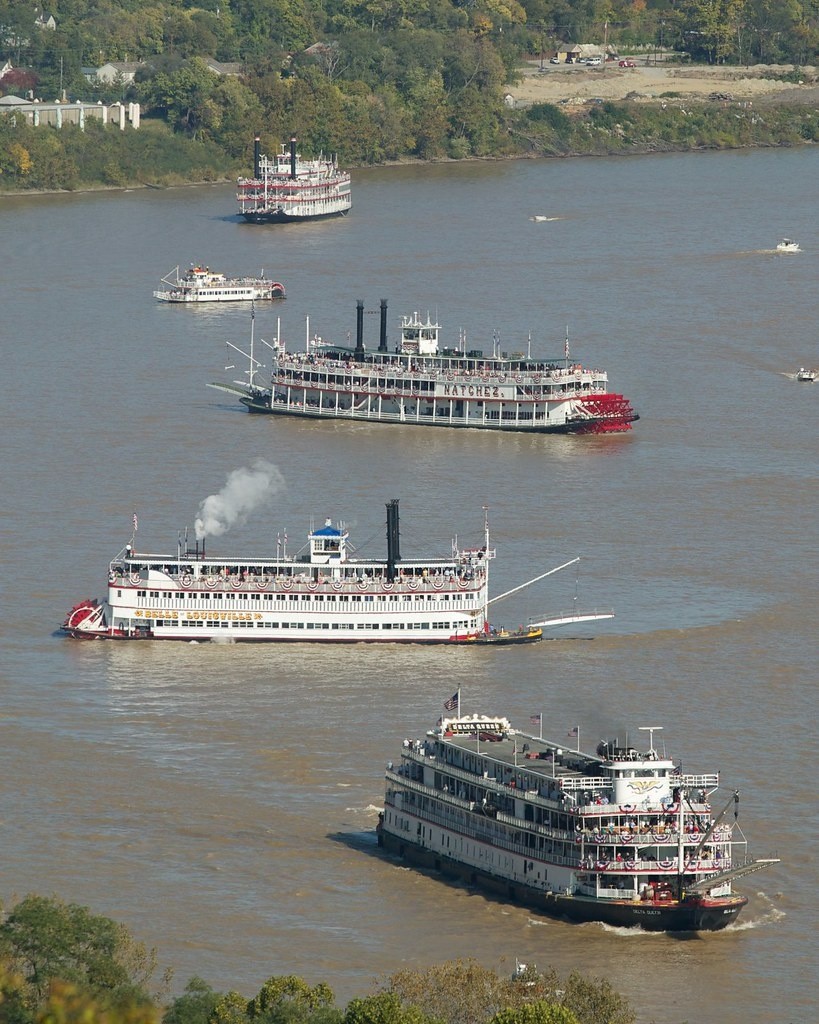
top-left (618, 61), bottom-right (637, 68)
top-left (550, 57), bottom-right (560, 64)
top-left (575, 57), bottom-right (586, 64)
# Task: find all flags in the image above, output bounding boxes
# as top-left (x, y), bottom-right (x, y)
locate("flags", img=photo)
top-left (444, 693), bottom-right (458, 712)
top-left (528, 714), bottom-right (540, 724)
top-left (278, 534), bottom-right (287, 546)
top-left (567, 727), bottom-right (578, 736)
top-left (565, 339), bottom-right (570, 357)
top-left (133, 513), bottom-right (138, 530)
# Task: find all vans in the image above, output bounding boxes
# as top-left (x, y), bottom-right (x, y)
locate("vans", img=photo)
top-left (586, 58), bottom-right (602, 67)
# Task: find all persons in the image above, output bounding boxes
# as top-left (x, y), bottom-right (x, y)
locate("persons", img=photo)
top-left (443, 784), bottom-right (448, 793)
top-left (685, 851), bottom-right (690, 861)
top-left (511, 776), bottom-right (515, 787)
top-left (489, 624), bottom-right (494, 633)
top-left (401, 737), bottom-right (429, 753)
top-left (697, 789), bottom-right (708, 803)
top-left (275, 342), bottom-right (606, 423)
top-left (378, 812), bottom-right (383, 824)
top-left (623, 818), bottom-right (715, 833)
top-left (399, 763), bottom-right (409, 774)
top-left (717, 850), bottom-right (728, 858)
top-left (597, 795), bottom-right (609, 804)
top-left (600, 852), bottom-right (631, 861)
top-left (165, 566), bottom-right (471, 583)
top-left (619, 880), bottom-right (625, 889)
top-left (330, 540), bottom-right (335, 547)
top-left (126, 543), bottom-right (131, 557)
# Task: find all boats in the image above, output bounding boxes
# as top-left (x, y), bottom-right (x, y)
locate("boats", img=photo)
top-left (776, 237), bottom-right (799, 252)
top-left (59, 496), bottom-right (619, 646)
top-left (151, 262), bottom-right (288, 304)
top-left (375, 682), bottom-right (783, 934)
top-left (236, 131), bottom-right (353, 224)
top-left (795, 367), bottom-right (817, 383)
top-left (204, 296), bottom-right (642, 436)
